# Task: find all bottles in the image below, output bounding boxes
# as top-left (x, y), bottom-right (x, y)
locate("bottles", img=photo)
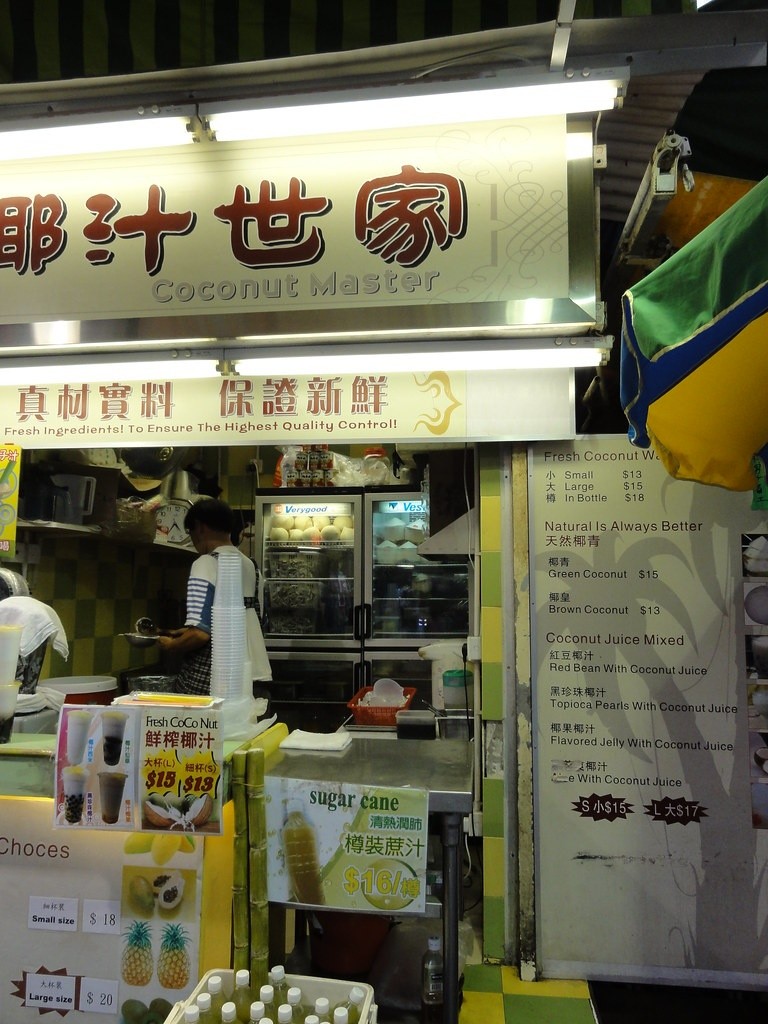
top-left (185, 965), bottom-right (364, 1024)
top-left (421, 937), bottom-right (444, 1004)
top-left (282, 800), bottom-right (327, 905)
top-left (19, 450), bottom-right (32, 519)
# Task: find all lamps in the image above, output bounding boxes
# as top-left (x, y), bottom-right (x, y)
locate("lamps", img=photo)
top-left (197, 64), bottom-right (631, 143)
top-left (0, 102), bottom-right (201, 163)
top-left (0, 334), bottom-right (614, 386)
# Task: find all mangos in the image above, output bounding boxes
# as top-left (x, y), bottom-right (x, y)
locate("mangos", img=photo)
top-left (124, 832), bottom-right (196, 866)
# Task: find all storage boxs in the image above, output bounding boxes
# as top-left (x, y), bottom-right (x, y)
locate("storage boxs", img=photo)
top-left (435, 709), bottom-right (474, 739)
top-left (346, 687), bottom-right (417, 726)
top-left (42, 459), bottom-right (121, 524)
top-left (163, 969), bottom-right (378, 1024)
top-left (395, 710), bottom-right (435, 740)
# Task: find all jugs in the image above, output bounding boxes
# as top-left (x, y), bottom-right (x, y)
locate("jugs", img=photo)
top-left (442, 669), bottom-right (474, 709)
top-left (50, 474), bottom-right (96, 524)
top-left (23, 465), bottom-right (72, 521)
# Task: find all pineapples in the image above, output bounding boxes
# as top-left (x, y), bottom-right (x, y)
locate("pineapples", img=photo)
top-left (120, 921), bottom-right (190, 989)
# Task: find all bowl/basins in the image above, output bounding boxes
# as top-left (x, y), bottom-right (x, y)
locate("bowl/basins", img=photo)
top-left (119, 633), bottom-right (160, 648)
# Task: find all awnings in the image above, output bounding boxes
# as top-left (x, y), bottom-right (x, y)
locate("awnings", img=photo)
top-left (620, 176), bottom-right (768, 491)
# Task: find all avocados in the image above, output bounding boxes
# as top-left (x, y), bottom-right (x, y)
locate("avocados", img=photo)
top-left (120, 998), bottom-right (173, 1024)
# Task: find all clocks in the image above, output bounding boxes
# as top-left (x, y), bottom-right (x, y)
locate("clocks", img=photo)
top-left (154, 499), bottom-right (194, 546)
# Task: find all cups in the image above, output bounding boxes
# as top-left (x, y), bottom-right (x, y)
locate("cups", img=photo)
top-left (67, 710), bottom-right (93, 765)
top-left (0, 625), bottom-right (23, 682)
top-left (97, 771), bottom-right (129, 824)
top-left (62, 766), bottom-right (90, 823)
top-left (0, 682), bottom-right (22, 745)
top-left (100, 710), bottom-right (129, 766)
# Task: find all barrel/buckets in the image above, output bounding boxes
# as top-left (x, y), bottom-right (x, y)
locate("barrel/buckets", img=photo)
top-left (308, 910), bottom-right (392, 977)
top-left (38, 676), bottom-right (118, 705)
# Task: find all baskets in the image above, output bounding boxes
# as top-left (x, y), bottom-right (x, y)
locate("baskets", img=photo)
top-left (347, 687), bottom-right (417, 726)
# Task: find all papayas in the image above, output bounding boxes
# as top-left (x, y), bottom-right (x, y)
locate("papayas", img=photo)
top-left (127, 869), bottom-right (185, 912)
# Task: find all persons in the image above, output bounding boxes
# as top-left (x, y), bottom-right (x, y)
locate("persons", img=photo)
top-left (158, 498), bottom-right (272, 695)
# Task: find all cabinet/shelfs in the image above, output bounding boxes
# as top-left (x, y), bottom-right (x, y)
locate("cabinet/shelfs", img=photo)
top-left (264, 734), bottom-right (474, 1024)
top-left (255, 442), bottom-right (478, 739)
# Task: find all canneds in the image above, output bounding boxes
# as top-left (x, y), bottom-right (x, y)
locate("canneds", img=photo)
top-left (287, 444), bottom-right (340, 487)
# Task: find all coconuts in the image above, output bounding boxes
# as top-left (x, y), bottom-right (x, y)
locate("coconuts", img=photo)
top-left (144, 791), bottom-right (213, 827)
top-left (376, 518), bottom-right (429, 564)
